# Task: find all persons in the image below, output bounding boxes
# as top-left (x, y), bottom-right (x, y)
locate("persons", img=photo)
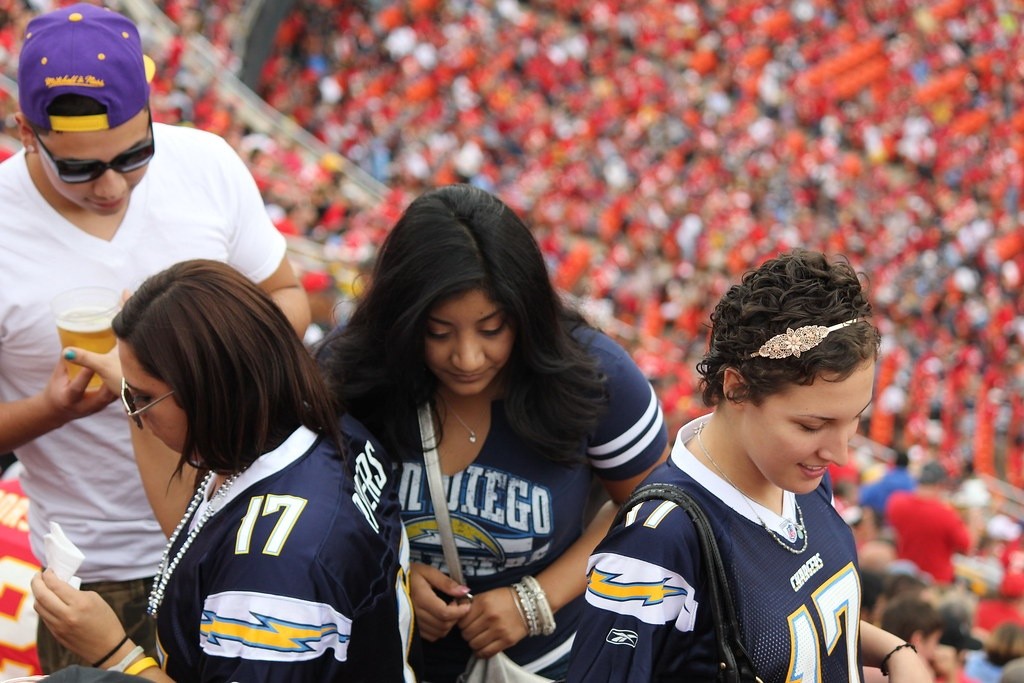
top-left (30, 258), bottom-right (416, 682)
top-left (312, 185), bottom-right (673, 682)
top-left (0, 0), bottom-right (1024, 680)
top-left (562, 251), bottom-right (931, 683)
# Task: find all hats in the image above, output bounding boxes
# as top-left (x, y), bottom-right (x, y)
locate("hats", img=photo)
top-left (882, 560), bottom-right (932, 586)
top-left (18, 2), bottom-right (155, 133)
top-left (938, 601), bottom-right (983, 650)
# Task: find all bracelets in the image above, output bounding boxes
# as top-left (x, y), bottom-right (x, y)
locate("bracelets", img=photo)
top-left (90, 636), bottom-right (158, 675)
top-left (510, 575), bottom-right (556, 638)
top-left (881, 642), bottom-right (919, 676)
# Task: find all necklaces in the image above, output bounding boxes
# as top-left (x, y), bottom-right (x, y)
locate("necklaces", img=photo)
top-left (694, 418), bottom-right (808, 555)
top-left (440, 394), bottom-right (491, 443)
top-left (147, 462), bottom-right (252, 616)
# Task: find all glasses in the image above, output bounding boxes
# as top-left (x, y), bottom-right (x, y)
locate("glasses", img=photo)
top-left (121, 376), bottom-right (174, 430)
top-left (29, 98), bottom-right (155, 184)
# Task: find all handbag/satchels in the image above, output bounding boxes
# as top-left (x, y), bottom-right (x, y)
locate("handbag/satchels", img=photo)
top-left (456, 651), bottom-right (556, 683)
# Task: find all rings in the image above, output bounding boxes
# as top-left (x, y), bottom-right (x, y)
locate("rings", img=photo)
top-left (465, 593), bottom-right (475, 601)
